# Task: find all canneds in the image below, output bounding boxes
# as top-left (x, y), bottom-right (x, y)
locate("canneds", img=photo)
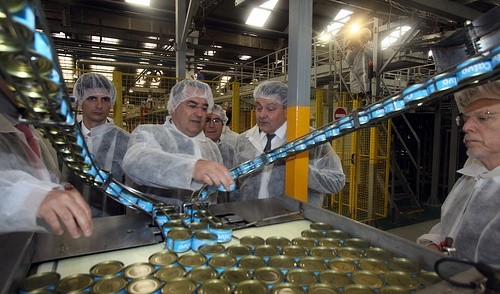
top-left (104, 181), bottom-right (178, 225)
top-left (351, 44), bottom-right (500, 126)
top-left (38, 112), bottom-right (110, 188)
top-left (14, 222), bottom-right (444, 294)
top-left (182, 161), bottom-right (255, 228)
top-left (251, 114), bottom-right (354, 169)
top-left (0, 0), bottom-right (68, 122)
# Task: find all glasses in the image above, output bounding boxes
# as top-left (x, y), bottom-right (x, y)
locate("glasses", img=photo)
top-left (456, 112), bottom-right (500, 126)
top-left (205, 119), bottom-right (222, 125)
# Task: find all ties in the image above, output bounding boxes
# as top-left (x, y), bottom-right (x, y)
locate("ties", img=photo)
top-left (263, 134), bottom-right (276, 152)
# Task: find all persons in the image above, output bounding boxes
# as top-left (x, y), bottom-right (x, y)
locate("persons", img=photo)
top-left (203, 105), bottom-right (242, 172)
top-left (233, 81), bottom-right (345, 209)
top-left (0, 101), bottom-right (94, 242)
top-left (72, 72), bottom-right (132, 181)
top-left (122, 77), bottom-right (235, 197)
top-left (414, 82), bottom-right (500, 271)
